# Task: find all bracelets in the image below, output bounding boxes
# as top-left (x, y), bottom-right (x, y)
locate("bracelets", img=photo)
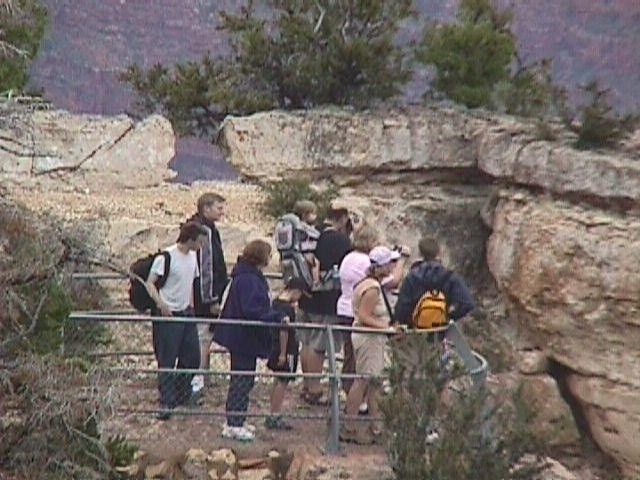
top-left (155, 302), bottom-right (168, 309)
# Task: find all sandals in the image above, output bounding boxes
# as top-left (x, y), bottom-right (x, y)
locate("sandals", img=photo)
top-left (300, 386), bottom-right (334, 407)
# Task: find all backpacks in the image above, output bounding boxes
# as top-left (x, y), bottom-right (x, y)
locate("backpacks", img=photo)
top-left (274, 213), bottom-right (313, 291)
top-left (129, 248), bottom-right (170, 312)
top-left (411, 286), bottom-right (449, 329)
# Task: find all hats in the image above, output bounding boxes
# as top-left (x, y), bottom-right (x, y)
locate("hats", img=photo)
top-left (369, 246), bottom-right (401, 266)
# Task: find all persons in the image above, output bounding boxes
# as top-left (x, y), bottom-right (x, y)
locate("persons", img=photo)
top-left (211, 238), bottom-right (292, 444)
top-left (145, 221), bottom-right (212, 424)
top-left (262, 276), bottom-right (308, 433)
top-left (179, 191), bottom-right (230, 394)
top-left (272, 197), bottom-right (475, 446)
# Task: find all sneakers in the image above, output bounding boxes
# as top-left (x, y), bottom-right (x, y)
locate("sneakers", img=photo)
top-left (221, 420), bottom-right (255, 440)
top-left (190, 374), bottom-right (205, 392)
top-left (264, 415), bottom-right (291, 430)
top-left (338, 424), bottom-right (373, 445)
